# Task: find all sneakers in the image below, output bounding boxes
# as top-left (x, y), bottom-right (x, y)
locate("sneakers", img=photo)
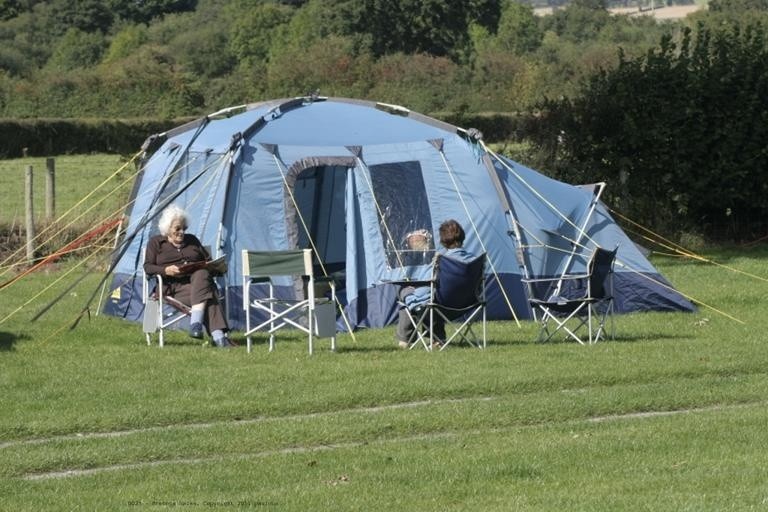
top-left (189, 322), bottom-right (203, 339)
top-left (210, 336), bottom-right (226, 347)
top-left (399, 341), bottom-right (446, 350)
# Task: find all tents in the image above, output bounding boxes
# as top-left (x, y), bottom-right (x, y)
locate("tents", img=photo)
top-left (100, 90), bottom-right (698, 345)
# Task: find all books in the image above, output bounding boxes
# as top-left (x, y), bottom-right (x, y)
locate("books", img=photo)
top-left (179, 253), bottom-right (227, 274)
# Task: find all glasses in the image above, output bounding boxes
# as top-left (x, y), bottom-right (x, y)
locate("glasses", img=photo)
top-left (174, 226), bottom-right (188, 232)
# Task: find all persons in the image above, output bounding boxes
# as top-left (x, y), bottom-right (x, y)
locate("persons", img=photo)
top-left (395, 219), bottom-right (477, 348)
top-left (141, 203), bottom-right (237, 346)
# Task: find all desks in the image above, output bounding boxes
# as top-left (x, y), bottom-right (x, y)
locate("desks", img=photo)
top-left (386, 278), bottom-right (433, 287)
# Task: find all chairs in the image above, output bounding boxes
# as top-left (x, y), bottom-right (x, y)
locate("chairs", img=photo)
top-left (143, 239), bottom-right (226, 347)
top-left (521, 244), bottom-right (617, 346)
top-left (241, 247), bottom-right (338, 354)
top-left (398, 252), bottom-right (489, 352)
